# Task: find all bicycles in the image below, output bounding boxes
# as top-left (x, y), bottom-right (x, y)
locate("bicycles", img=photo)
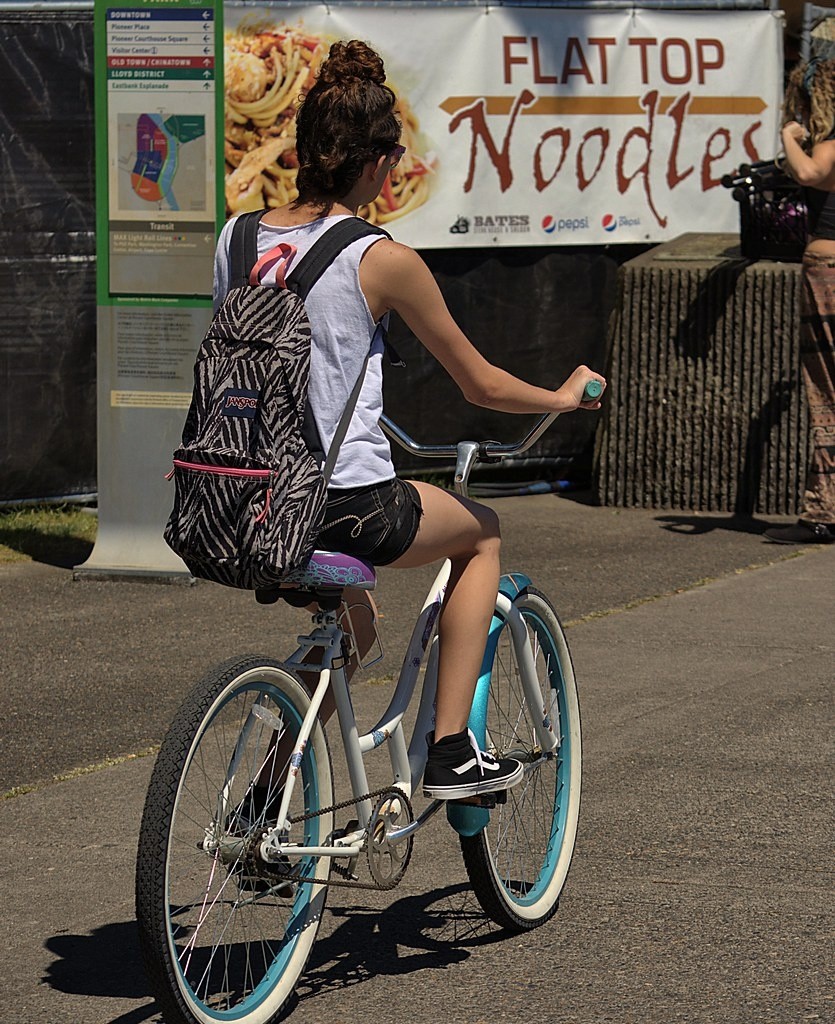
top-left (135, 381), bottom-right (583, 1023)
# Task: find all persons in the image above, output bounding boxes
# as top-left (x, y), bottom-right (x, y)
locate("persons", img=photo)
top-left (211, 39), bottom-right (613, 894)
top-left (782, 50), bottom-right (835, 531)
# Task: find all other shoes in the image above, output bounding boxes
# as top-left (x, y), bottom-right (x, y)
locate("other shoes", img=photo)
top-left (423, 727), bottom-right (523, 801)
top-left (227, 791), bottom-right (297, 897)
top-left (763, 518), bottom-right (835, 543)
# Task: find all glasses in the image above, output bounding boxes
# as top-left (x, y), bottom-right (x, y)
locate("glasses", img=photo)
top-left (378, 143), bottom-right (406, 169)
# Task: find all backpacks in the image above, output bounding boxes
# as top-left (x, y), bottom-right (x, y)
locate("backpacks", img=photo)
top-left (162, 208), bottom-right (396, 590)
